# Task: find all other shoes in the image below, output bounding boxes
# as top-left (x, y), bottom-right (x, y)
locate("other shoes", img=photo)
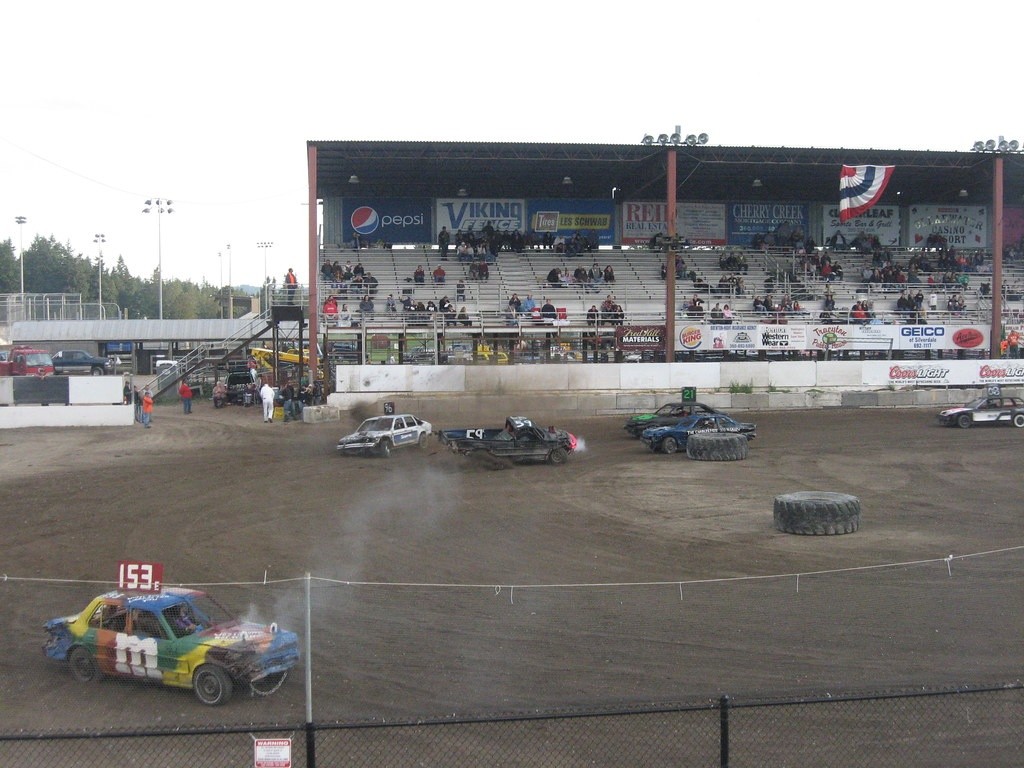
top-left (145, 426), bottom-right (150, 428)
top-left (188, 411), bottom-right (192, 413)
top-left (185, 412), bottom-right (189, 414)
top-left (268, 418), bottom-right (273, 423)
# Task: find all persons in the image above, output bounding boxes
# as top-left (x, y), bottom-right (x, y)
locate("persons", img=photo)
top-left (699, 417), bottom-right (714, 428)
top-left (672, 407), bottom-right (688, 418)
top-left (316, 218), bottom-right (1024, 364)
top-left (124, 365), bottom-right (321, 428)
top-left (986, 399), bottom-right (995, 409)
top-left (168, 604), bottom-right (198, 635)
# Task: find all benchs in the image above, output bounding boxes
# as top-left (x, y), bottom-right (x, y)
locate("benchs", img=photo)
top-left (319, 246), bottom-right (1024, 335)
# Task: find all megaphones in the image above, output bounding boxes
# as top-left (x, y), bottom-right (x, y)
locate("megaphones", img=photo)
top-left (642, 136), bottom-right (654, 145)
top-left (985, 140), bottom-right (996, 150)
top-left (658, 134), bottom-right (669, 145)
top-left (998, 140), bottom-right (1009, 152)
top-left (670, 133), bottom-right (681, 145)
top-left (697, 133), bottom-right (709, 145)
top-left (1008, 140), bottom-right (1019, 151)
top-left (973, 141), bottom-right (985, 152)
top-left (685, 135), bottom-right (697, 145)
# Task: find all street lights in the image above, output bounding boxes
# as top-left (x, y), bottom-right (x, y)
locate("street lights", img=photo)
top-left (15, 216), bottom-right (26, 321)
top-left (642, 133), bottom-right (709, 361)
top-left (218, 253), bottom-right (223, 319)
top-left (257, 242), bottom-right (273, 319)
top-left (227, 244), bottom-right (231, 318)
top-left (975, 139), bottom-right (1019, 359)
top-left (94, 233), bottom-right (106, 320)
top-left (143, 198), bottom-right (174, 320)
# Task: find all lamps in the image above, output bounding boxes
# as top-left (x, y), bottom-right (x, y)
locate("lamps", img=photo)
top-left (347, 175), bottom-right (362, 186)
top-left (959, 189), bottom-right (969, 197)
top-left (753, 179), bottom-right (763, 187)
top-left (457, 189), bottom-right (471, 197)
top-left (562, 176), bottom-right (573, 185)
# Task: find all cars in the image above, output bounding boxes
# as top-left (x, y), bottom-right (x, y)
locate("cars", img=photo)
top-left (42, 587), bottom-right (301, 706)
top-left (641, 414), bottom-right (756, 454)
top-left (438, 417), bottom-right (578, 471)
top-left (336, 414), bottom-right (432, 458)
top-left (624, 402), bottom-right (730, 438)
top-left (51, 350), bottom-right (114, 377)
top-left (224, 368), bottom-right (262, 402)
top-left (333, 343), bottom-right (585, 363)
top-left (938, 396), bottom-right (1024, 429)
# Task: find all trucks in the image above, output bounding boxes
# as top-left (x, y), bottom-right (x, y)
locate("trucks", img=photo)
top-left (0, 346), bottom-right (56, 376)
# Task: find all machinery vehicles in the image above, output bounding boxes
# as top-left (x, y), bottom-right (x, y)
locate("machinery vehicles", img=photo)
top-left (252, 348), bottom-right (324, 379)
